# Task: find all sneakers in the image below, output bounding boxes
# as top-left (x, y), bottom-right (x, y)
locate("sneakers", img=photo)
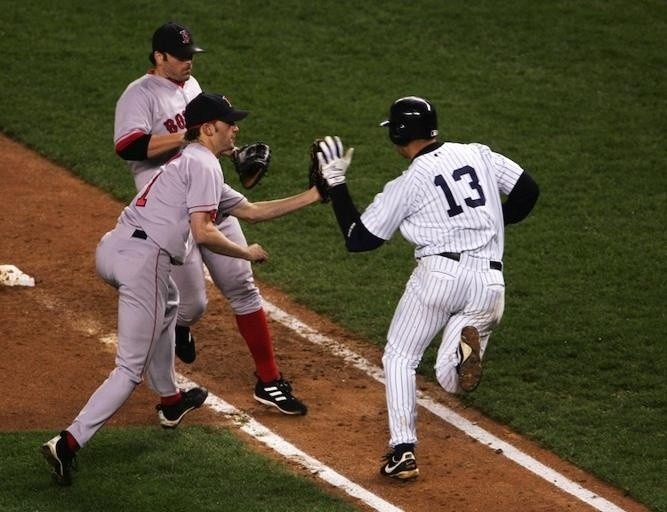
top-left (455, 325), bottom-right (483, 395)
top-left (380, 450), bottom-right (421, 481)
top-left (39, 430), bottom-right (73, 489)
top-left (253, 370), bottom-right (308, 417)
top-left (175, 324), bottom-right (197, 364)
top-left (156, 386), bottom-right (208, 431)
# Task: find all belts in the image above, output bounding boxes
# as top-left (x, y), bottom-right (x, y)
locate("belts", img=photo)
top-left (132, 229), bottom-right (147, 240)
top-left (438, 252), bottom-right (503, 272)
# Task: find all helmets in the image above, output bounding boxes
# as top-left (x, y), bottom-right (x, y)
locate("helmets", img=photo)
top-left (378, 95), bottom-right (440, 146)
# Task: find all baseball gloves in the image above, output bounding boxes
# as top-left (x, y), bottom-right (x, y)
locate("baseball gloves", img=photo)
top-left (307, 138), bottom-right (341, 203)
top-left (231, 141), bottom-right (271, 191)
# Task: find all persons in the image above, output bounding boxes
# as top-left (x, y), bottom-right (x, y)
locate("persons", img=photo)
top-left (42, 93), bottom-right (323, 487)
top-left (316, 95), bottom-right (540, 481)
top-left (113, 23), bottom-right (308, 416)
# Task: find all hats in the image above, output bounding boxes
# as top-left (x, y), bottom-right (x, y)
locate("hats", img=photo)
top-left (151, 21), bottom-right (207, 55)
top-left (183, 92), bottom-right (250, 129)
